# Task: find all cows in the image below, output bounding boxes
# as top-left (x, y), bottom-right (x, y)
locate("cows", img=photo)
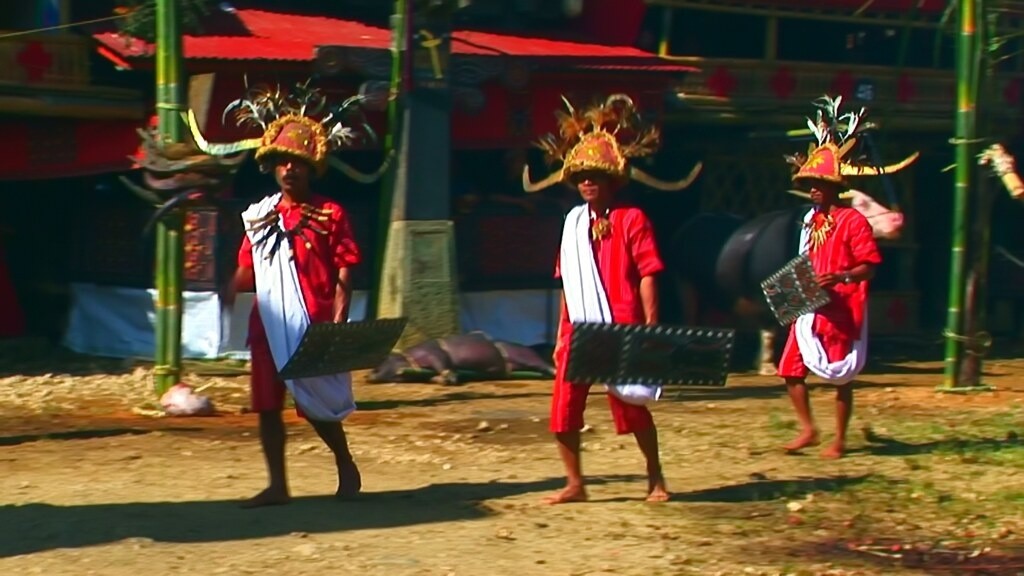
top-left (365, 333), bottom-right (556, 385)
top-left (675, 188), bottom-right (903, 377)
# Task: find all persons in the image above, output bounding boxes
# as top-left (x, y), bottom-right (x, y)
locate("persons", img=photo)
top-left (540, 131), bottom-right (669, 504)
top-left (774, 142), bottom-right (882, 460)
top-left (232, 114), bottom-right (362, 508)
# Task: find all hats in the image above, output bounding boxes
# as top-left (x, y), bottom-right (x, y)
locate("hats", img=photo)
top-left (785, 92), bottom-right (921, 198)
top-left (179, 73), bottom-right (398, 193)
top-left (522, 89), bottom-right (704, 195)
